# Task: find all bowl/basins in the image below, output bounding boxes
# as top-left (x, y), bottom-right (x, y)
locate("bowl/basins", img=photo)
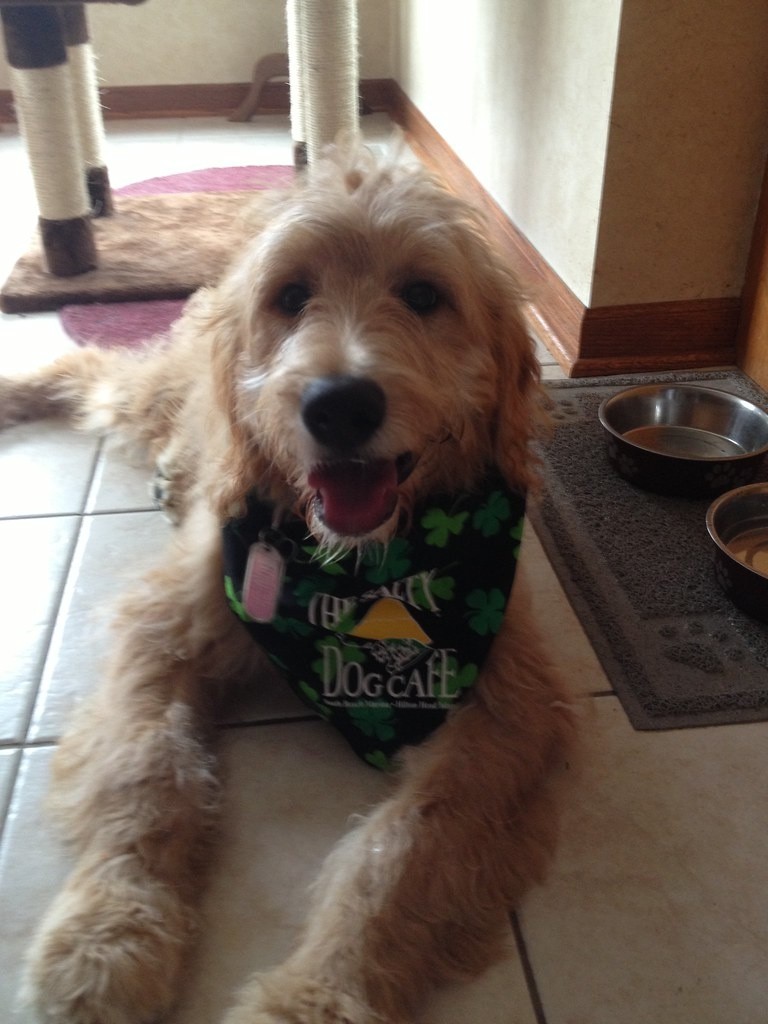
top-left (704, 481), bottom-right (768, 624)
top-left (598, 383), bottom-right (768, 501)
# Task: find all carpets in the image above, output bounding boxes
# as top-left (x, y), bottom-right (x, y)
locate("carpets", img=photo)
top-left (522, 362), bottom-right (768, 732)
top-left (58, 159), bottom-right (299, 346)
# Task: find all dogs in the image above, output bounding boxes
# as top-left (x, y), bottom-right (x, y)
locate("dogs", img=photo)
top-left (0, 128), bottom-right (587, 1022)
